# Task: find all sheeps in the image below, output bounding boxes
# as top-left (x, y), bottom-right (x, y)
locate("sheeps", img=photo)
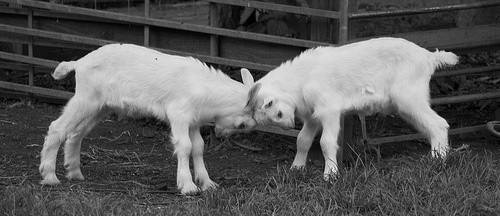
top-left (252, 37), bottom-right (460, 185)
top-left (38, 43), bottom-right (262, 196)
